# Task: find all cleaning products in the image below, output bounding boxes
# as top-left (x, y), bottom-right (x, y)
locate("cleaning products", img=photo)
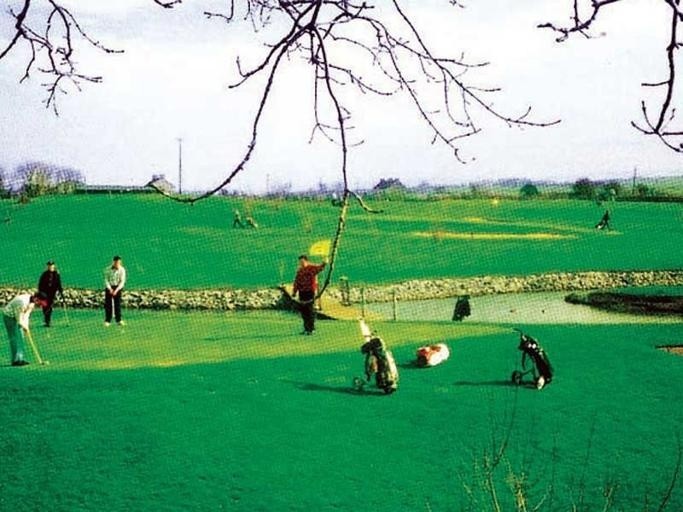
top-left (353, 329), bottom-right (400, 395)
top-left (512, 328), bottom-right (554, 390)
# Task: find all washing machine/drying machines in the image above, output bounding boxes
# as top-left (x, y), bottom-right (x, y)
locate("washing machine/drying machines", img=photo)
top-left (63, 303), bottom-right (71, 326)
top-left (112, 296), bottom-right (115, 317)
top-left (27, 331), bottom-right (50, 364)
top-left (364, 329), bottom-right (378, 341)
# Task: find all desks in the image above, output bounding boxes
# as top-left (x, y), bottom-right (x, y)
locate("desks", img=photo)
top-left (174, 136), bottom-right (182, 193)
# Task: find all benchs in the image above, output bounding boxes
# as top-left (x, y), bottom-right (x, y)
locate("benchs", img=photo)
top-left (308, 238), bottom-right (331, 259)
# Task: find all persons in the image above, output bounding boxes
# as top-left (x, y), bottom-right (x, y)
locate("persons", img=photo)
top-left (598, 208), bottom-right (613, 233)
top-left (0, 291), bottom-right (48, 368)
top-left (290, 253), bottom-right (328, 336)
top-left (338, 192), bottom-right (344, 211)
top-left (101, 255), bottom-right (128, 326)
top-left (243, 213), bottom-right (258, 230)
top-left (329, 188), bottom-right (337, 208)
top-left (35, 259), bottom-right (66, 330)
top-left (229, 208), bottom-right (244, 229)
